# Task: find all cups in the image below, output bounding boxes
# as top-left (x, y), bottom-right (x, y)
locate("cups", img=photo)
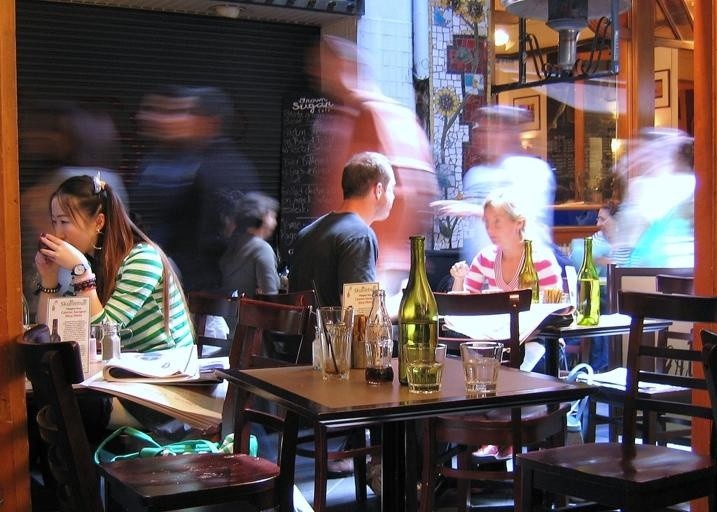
top-left (38, 233), bottom-right (55, 257)
top-left (459, 342), bottom-right (504, 395)
top-left (315, 307), bottom-right (354, 383)
top-left (352, 336), bottom-right (368, 369)
top-left (90, 324), bottom-right (133, 354)
top-left (403, 342), bottom-right (448, 395)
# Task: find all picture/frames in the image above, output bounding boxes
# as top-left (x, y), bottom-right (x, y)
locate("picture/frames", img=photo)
top-left (513, 95), bottom-right (540, 131)
top-left (654, 69), bottom-right (671, 109)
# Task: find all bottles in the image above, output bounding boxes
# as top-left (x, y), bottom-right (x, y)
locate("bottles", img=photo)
top-left (518, 239), bottom-right (540, 305)
top-left (311, 321), bottom-right (347, 371)
top-left (101, 323), bottom-right (121, 363)
top-left (50, 319), bottom-right (61, 343)
top-left (398, 235), bottom-right (440, 387)
top-left (575, 236), bottom-right (601, 327)
top-left (22, 292), bottom-right (30, 330)
top-left (90, 335), bottom-right (97, 364)
top-left (364, 290), bottom-right (394, 385)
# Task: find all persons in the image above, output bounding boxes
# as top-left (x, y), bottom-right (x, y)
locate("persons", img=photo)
top-left (20, 36), bottom-right (695, 512)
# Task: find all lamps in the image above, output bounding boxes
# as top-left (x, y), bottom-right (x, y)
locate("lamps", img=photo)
top-left (499, 0), bottom-right (629, 79)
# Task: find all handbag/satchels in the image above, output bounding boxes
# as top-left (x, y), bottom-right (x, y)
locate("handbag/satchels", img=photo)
top-left (567, 414), bottom-right (584, 445)
top-left (146, 432), bottom-right (257, 456)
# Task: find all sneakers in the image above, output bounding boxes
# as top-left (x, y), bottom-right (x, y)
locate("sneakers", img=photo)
top-left (328, 450), bottom-right (372, 478)
top-left (473, 444), bottom-right (513, 461)
